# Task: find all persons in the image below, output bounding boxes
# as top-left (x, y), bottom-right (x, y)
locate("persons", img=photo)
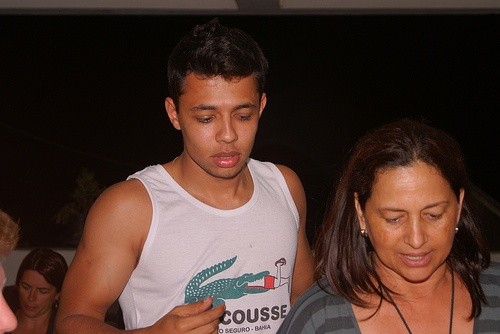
top-left (276, 122), bottom-right (500, 334)
top-left (0, 212), bottom-right (66, 334)
top-left (53, 36), bottom-right (316, 334)
